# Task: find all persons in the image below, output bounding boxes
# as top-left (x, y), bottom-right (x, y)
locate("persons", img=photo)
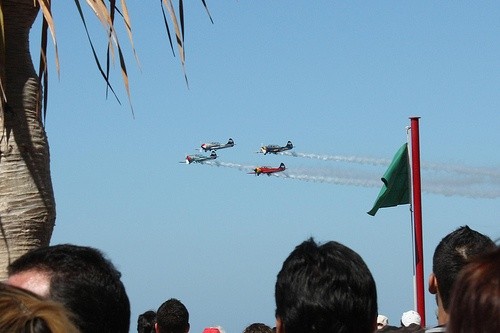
top-left (6, 244), bottom-right (131, 333)
top-left (392, 310), bottom-right (426, 332)
top-left (425, 224), bottom-right (498, 333)
top-left (275, 239), bottom-right (379, 333)
top-left (137, 311), bottom-right (158, 333)
top-left (375, 315), bottom-right (389, 333)
top-left (154, 299), bottom-right (190, 333)
top-left (0, 282), bottom-right (81, 333)
top-left (446, 249), bottom-right (500, 333)
top-left (242, 323), bottom-right (275, 333)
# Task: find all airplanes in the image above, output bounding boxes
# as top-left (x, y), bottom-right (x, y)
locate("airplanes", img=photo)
top-left (185, 150), bottom-right (217, 165)
top-left (200, 137), bottom-right (235, 152)
top-left (256, 140), bottom-right (294, 155)
top-left (246, 162), bottom-right (286, 177)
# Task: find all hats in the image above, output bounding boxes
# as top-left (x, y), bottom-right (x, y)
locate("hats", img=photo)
top-left (402, 310), bottom-right (421, 327)
top-left (377, 315), bottom-right (389, 325)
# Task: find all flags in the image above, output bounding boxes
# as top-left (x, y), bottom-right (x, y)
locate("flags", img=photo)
top-left (366, 142), bottom-right (411, 217)
